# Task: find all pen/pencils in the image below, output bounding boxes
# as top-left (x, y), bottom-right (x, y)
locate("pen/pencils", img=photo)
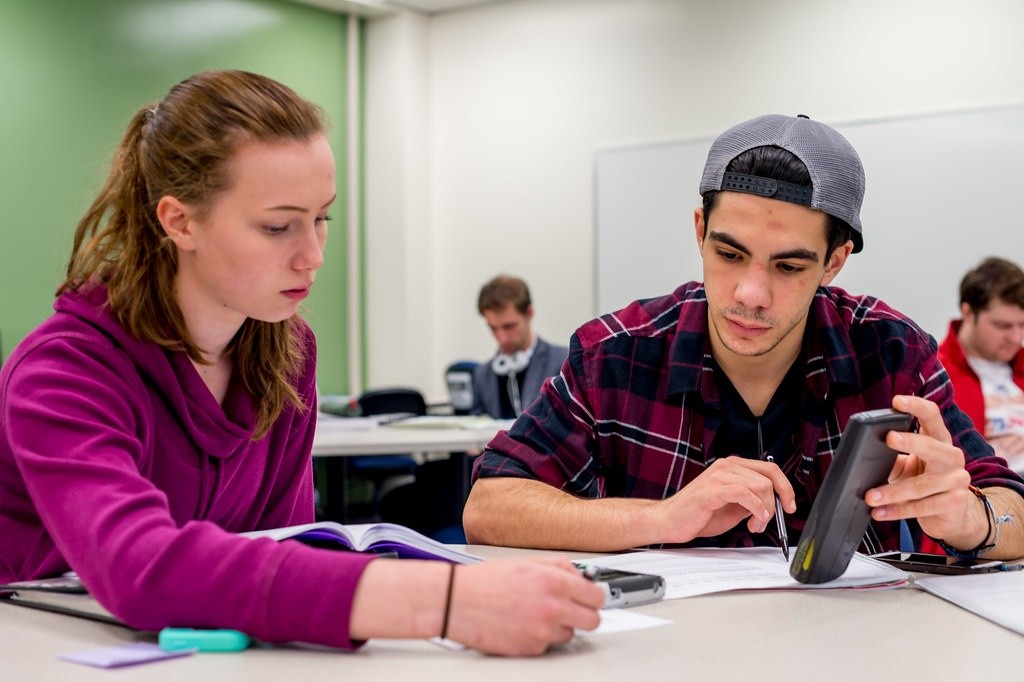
top-left (766, 455), bottom-right (790, 563)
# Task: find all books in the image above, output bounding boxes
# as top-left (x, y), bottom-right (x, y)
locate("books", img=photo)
top-left (0, 521), bottom-right (483, 631)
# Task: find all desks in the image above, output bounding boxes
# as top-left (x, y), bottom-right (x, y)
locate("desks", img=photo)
top-left (0, 544), bottom-right (1024, 682)
top-left (309, 421), bottom-right (520, 543)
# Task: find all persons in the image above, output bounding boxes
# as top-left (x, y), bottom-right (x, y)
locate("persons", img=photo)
top-left (0, 69), bottom-right (605, 657)
top-left (472, 277), bottom-right (569, 421)
top-left (462, 113), bottom-right (1024, 561)
top-left (919, 258), bottom-right (1024, 555)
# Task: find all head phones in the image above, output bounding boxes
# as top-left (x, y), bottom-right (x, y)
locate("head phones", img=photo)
top-left (491, 337), bottom-right (538, 376)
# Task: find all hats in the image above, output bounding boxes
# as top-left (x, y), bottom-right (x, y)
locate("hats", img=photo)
top-left (697, 113), bottom-right (864, 256)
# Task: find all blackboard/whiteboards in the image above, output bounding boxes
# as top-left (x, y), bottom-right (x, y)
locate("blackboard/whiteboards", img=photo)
top-left (590, 102), bottom-right (1024, 349)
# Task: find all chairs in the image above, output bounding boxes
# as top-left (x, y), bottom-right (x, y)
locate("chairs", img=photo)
top-left (327, 362), bottom-right (482, 544)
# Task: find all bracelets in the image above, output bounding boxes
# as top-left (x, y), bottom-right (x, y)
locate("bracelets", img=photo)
top-left (441, 563), bottom-right (455, 639)
top-left (938, 484), bottom-right (999, 558)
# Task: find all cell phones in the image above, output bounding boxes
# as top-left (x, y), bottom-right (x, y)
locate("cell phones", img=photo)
top-left (159, 626), bottom-right (252, 654)
top-left (868, 550), bottom-right (1004, 570)
